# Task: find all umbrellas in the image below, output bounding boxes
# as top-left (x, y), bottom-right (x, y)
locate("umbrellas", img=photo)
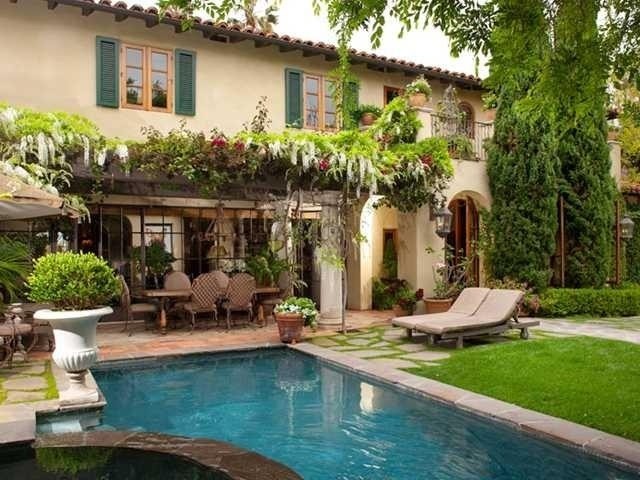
top-left (0, 173), bottom-right (65, 223)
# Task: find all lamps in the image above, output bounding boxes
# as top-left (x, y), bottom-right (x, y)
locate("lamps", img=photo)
top-left (430, 194), bottom-right (453, 237)
top-left (617, 198), bottom-right (634, 243)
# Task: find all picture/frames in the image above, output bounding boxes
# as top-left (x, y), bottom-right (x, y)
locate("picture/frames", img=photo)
top-left (145, 223), bottom-right (173, 255)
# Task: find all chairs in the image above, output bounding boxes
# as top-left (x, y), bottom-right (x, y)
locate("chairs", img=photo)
top-left (116, 272), bottom-right (299, 335)
top-left (392, 287), bottom-right (540, 347)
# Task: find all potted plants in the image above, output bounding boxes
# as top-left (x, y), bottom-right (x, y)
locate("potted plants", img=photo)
top-left (353, 73), bottom-right (432, 143)
top-left (273, 302), bottom-right (308, 342)
top-left (423, 239), bottom-right (481, 314)
top-left (23, 250), bottom-right (130, 392)
